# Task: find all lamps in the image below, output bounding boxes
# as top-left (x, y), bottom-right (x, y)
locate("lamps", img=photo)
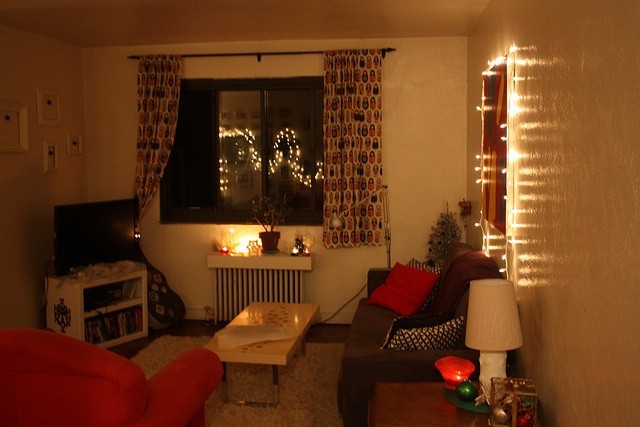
top-left (464, 279), bottom-right (525, 406)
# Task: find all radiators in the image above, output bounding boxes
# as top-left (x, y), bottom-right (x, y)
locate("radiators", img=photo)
top-left (207, 255), bottom-right (312, 327)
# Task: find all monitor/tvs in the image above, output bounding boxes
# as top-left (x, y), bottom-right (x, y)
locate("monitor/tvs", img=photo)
top-left (53, 197), bottom-right (139, 278)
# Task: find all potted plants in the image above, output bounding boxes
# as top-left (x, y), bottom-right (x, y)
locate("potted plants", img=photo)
top-left (249, 193), bottom-right (290, 255)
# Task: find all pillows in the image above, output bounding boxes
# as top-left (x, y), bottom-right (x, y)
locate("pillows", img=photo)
top-left (402, 258), bottom-right (442, 313)
top-left (367, 262), bottom-right (432, 315)
top-left (380, 315), bottom-right (456, 347)
top-left (389, 318), bottom-right (462, 352)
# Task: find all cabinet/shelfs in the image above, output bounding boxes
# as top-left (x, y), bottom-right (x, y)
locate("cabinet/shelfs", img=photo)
top-left (45, 271), bottom-right (149, 348)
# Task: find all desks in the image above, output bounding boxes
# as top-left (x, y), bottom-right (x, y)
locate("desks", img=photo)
top-left (367, 382), bottom-right (542, 427)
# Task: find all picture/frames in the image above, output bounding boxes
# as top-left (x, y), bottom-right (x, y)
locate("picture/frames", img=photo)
top-left (35, 89), bottom-right (62, 125)
top-left (42, 142), bottom-right (58, 172)
top-left (67, 133), bottom-right (82, 156)
top-left (0, 101), bottom-right (30, 154)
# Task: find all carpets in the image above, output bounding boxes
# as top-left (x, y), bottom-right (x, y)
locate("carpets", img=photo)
top-left (132, 334), bottom-right (348, 427)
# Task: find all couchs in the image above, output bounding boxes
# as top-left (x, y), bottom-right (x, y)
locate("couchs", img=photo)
top-left (338, 242), bottom-right (510, 427)
top-left (0, 325), bottom-right (225, 427)
